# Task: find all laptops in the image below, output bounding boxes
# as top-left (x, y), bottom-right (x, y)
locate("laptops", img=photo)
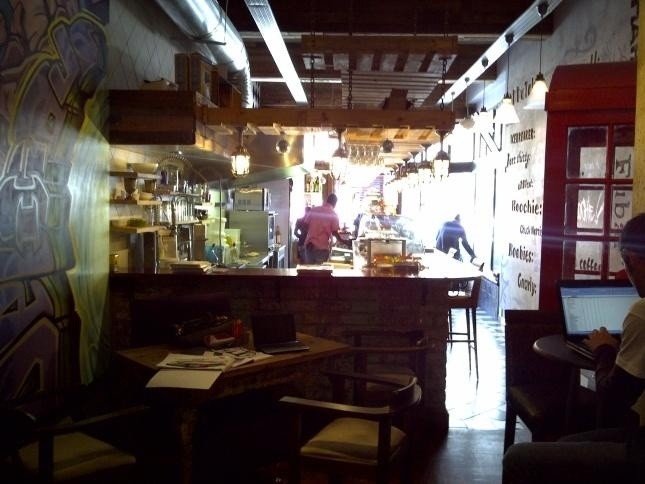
top-left (253, 309), bottom-right (311, 355)
top-left (556, 280), bottom-right (645, 362)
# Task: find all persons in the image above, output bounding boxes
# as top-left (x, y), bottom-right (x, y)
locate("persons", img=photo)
top-left (293, 205), bottom-right (311, 262)
top-left (299, 192), bottom-right (353, 264)
top-left (435, 212), bottom-right (477, 261)
top-left (502, 211), bottom-right (645, 483)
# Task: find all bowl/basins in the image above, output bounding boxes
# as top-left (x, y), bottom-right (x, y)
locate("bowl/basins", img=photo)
top-left (128, 163), bottom-right (159, 174)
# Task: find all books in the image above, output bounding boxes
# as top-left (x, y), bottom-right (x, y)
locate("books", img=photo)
top-left (295, 263), bottom-right (335, 274)
top-left (170, 259), bottom-right (211, 274)
top-left (144, 345), bottom-right (274, 390)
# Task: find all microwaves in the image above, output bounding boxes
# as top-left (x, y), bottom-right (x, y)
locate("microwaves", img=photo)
top-left (235, 188), bottom-right (272, 210)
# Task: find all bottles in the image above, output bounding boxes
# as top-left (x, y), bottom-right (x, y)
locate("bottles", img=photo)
top-left (275, 225), bottom-right (281, 244)
top-left (229, 246), bottom-right (239, 265)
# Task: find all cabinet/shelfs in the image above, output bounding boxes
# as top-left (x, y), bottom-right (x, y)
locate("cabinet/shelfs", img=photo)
top-left (109, 170), bottom-right (214, 268)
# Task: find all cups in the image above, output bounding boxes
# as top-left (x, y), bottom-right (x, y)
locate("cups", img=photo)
top-left (123, 174), bottom-right (138, 202)
top-left (144, 179), bottom-right (157, 193)
